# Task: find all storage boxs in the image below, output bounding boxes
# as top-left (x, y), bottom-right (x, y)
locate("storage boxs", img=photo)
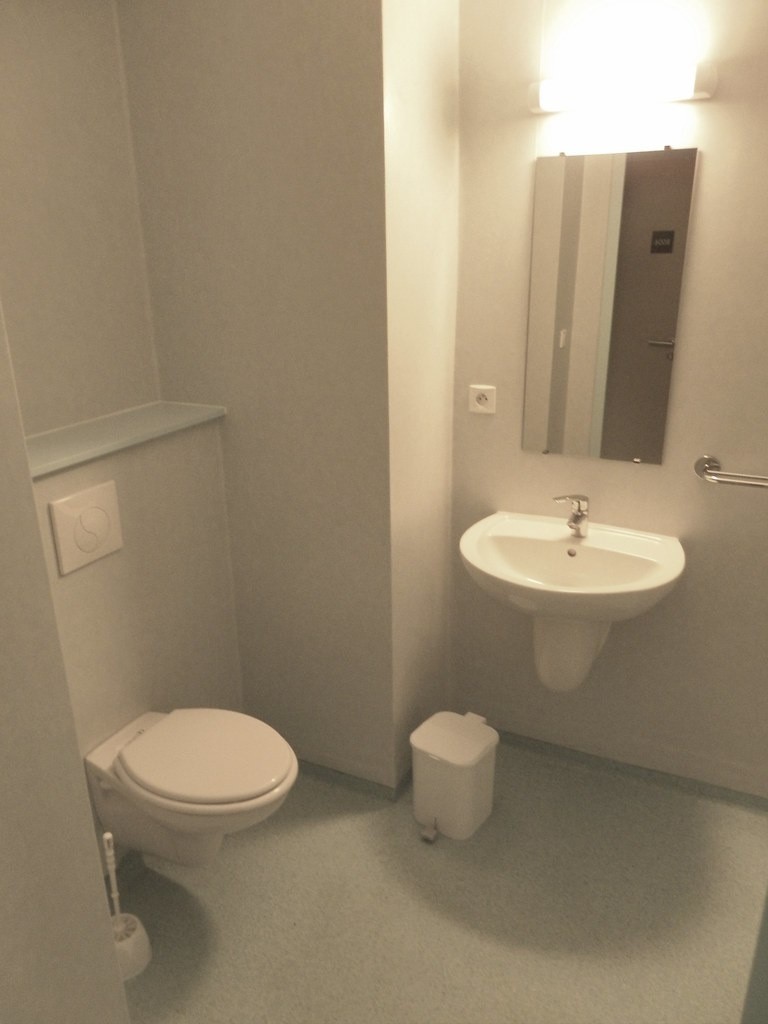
top-left (409, 711), bottom-right (500, 843)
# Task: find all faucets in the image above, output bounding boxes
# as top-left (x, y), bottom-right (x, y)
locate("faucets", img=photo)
top-left (553, 495), bottom-right (594, 538)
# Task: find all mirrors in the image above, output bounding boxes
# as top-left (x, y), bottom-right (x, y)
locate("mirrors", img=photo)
top-left (519, 147), bottom-right (695, 465)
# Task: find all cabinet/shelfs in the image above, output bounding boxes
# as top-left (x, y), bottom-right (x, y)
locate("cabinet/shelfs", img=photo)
top-left (19, 404), bottom-right (225, 875)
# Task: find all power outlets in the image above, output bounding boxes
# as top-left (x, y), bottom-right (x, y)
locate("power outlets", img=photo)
top-left (467, 386), bottom-right (496, 414)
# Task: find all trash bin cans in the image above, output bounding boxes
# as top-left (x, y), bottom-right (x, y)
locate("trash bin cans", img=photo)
top-left (402, 708), bottom-right (501, 842)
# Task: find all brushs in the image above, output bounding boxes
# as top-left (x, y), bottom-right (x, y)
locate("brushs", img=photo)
top-left (96, 830), bottom-right (154, 985)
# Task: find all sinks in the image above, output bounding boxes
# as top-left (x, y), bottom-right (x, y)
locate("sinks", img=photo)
top-left (458, 510), bottom-right (686, 625)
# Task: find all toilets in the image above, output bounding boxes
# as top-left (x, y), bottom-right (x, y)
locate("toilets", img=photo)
top-left (83, 699), bottom-right (300, 876)
